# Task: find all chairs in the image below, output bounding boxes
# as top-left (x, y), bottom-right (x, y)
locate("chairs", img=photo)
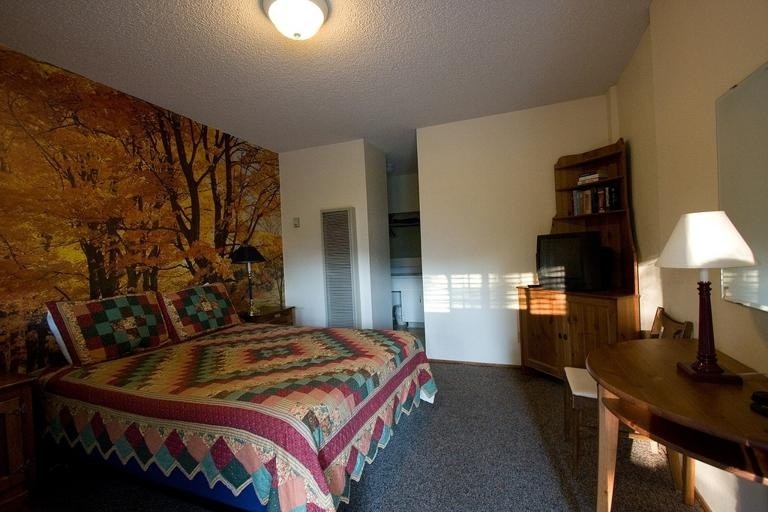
top-left (563, 307), bottom-right (694, 490)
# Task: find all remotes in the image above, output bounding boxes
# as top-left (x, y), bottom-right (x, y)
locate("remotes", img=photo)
top-left (527, 285), bottom-right (540, 288)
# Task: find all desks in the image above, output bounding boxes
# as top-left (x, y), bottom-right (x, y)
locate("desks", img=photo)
top-left (586, 338), bottom-right (768, 512)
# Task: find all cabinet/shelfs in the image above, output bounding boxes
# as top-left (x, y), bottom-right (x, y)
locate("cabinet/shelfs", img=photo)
top-left (516, 138), bottom-right (639, 380)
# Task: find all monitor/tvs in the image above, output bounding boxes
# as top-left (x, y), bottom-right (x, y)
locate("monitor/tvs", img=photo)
top-left (536, 231), bottom-right (617, 292)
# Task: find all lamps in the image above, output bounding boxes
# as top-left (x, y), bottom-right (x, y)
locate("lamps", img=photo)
top-left (232, 245), bottom-right (266, 314)
top-left (655, 211), bottom-right (758, 385)
top-left (262, 0), bottom-right (329, 41)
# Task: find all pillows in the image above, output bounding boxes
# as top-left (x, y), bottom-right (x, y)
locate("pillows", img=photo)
top-left (45, 282), bottom-right (240, 366)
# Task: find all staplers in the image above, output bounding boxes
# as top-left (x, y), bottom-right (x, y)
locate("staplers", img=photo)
top-left (749, 391), bottom-right (768, 417)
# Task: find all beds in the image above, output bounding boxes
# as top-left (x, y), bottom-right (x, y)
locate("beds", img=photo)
top-left (33, 323), bottom-right (438, 512)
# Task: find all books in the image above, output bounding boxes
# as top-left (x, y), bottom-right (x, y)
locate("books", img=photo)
top-left (572, 173), bottom-right (620, 215)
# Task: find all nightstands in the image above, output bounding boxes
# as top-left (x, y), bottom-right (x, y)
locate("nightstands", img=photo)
top-left (0, 374), bottom-right (39, 512)
top-left (238, 306), bottom-right (296, 326)
